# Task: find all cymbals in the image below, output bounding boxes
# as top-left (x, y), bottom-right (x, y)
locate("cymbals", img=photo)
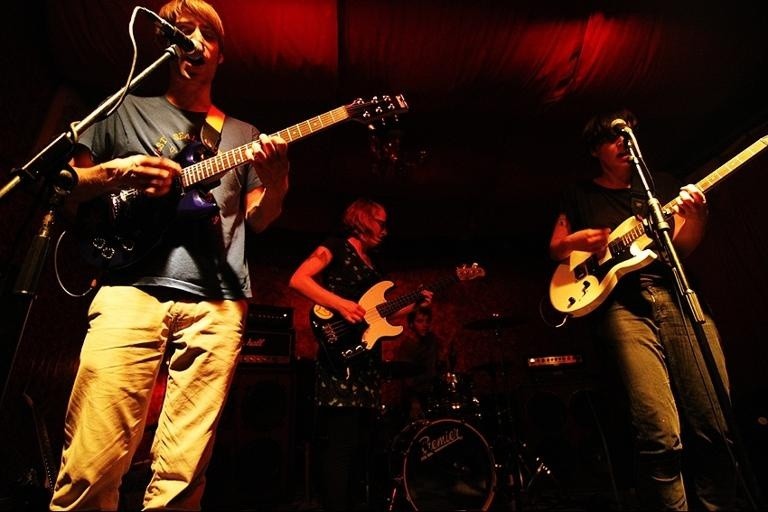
top-left (381, 359), bottom-right (425, 379)
top-left (465, 312), bottom-right (532, 329)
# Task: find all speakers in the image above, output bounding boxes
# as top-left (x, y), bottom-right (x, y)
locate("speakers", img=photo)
top-left (517, 378), bottom-right (631, 490)
top-left (229, 366), bottom-right (303, 504)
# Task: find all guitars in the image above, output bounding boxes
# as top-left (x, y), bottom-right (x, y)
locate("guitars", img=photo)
top-left (549, 134), bottom-right (768, 317)
top-left (310, 262), bottom-right (486, 367)
top-left (54, 94), bottom-right (409, 286)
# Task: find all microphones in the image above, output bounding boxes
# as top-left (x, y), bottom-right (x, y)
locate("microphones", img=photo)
top-left (611, 118), bottom-right (643, 160)
top-left (144, 8), bottom-right (204, 60)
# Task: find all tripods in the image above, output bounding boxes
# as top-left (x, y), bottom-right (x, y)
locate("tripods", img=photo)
top-left (487, 328), bottom-right (552, 510)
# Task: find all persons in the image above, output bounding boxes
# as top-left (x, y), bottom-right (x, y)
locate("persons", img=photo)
top-left (48, 0), bottom-right (289, 512)
top-left (288, 196), bottom-right (434, 512)
top-left (548, 110), bottom-right (739, 512)
top-left (397, 306), bottom-right (456, 390)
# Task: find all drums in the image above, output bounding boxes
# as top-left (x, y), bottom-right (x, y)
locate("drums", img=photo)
top-left (403, 417), bottom-right (499, 512)
top-left (427, 371), bottom-right (482, 423)
